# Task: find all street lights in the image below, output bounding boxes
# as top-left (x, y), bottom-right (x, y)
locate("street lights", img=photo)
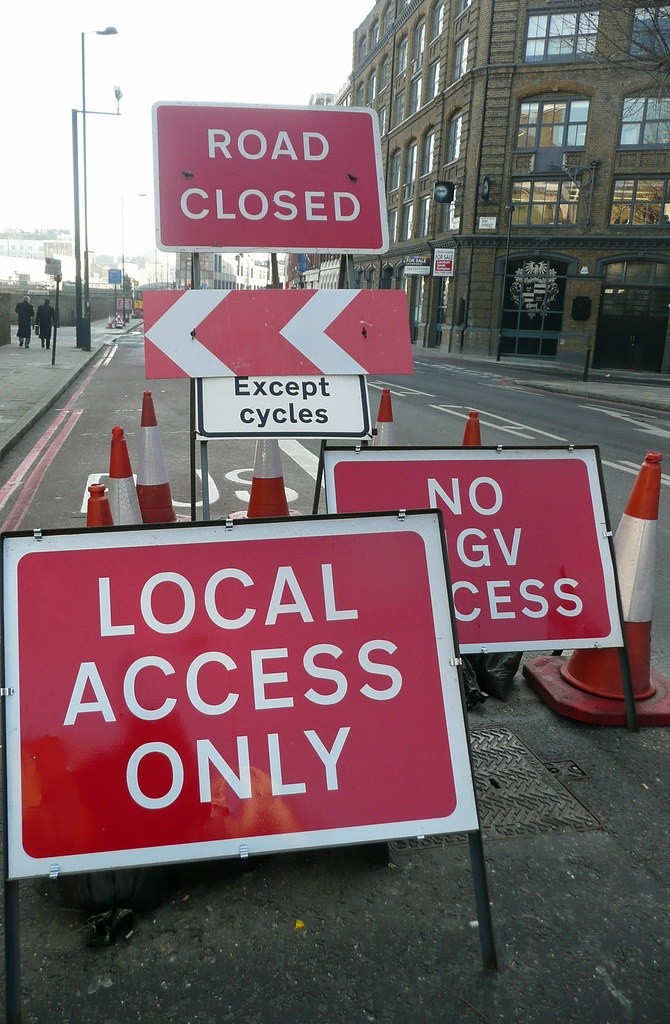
top-left (77, 24), bottom-right (119, 351)
top-left (70, 85), bottom-right (125, 347)
top-left (499, 204), bottom-right (516, 331)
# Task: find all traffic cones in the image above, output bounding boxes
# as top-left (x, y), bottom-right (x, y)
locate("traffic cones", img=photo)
top-left (461, 410), bottom-right (483, 448)
top-left (242, 439), bottom-right (294, 518)
top-left (516, 450), bottom-right (670, 728)
top-left (104, 314), bottom-right (114, 329)
top-left (83, 480), bottom-right (116, 525)
top-left (369, 385), bottom-right (398, 446)
top-left (132, 388), bottom-right (179, 523)
top-left (102, 423), bottom-right (145, 526)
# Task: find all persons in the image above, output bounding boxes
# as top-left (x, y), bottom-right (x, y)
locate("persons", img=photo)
top-left (35, 299), bottom-right (55, 349)
top-left (15, 296), bottom-right (34, 348)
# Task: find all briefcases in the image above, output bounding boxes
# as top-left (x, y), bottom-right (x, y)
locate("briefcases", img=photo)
top-left (35, 324), bottom-right (39, 335)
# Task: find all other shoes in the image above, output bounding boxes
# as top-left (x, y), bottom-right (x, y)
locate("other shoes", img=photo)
top-left (20, 341), bottom-right (23, 346)
top-left (25, 346), bottom-right (30, 348)
top-left (47, 347), bottom-right (50, 349)
top-left (42, 341), bottom-right (44, 348)
